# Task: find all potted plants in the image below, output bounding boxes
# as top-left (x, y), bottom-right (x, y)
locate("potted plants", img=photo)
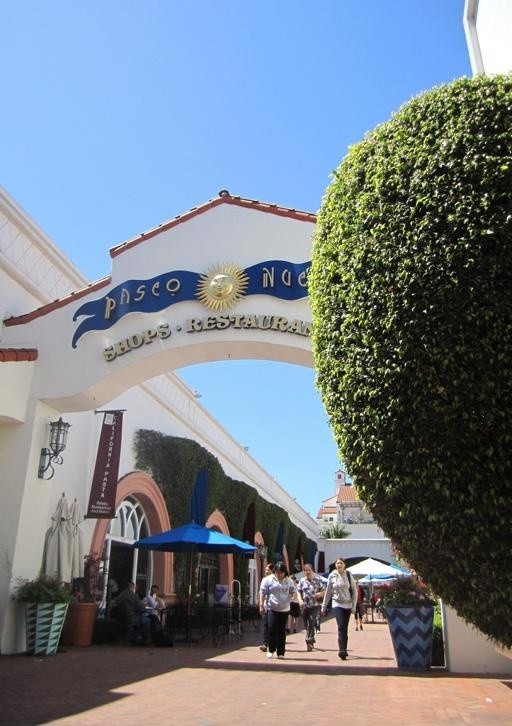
top-left (9, 568), bottom-right (81, 657)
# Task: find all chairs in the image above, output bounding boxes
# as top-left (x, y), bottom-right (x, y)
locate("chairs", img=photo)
top-left (168, 604), bottom-right (261, 648)
top-left (109, 602), bottom-right (132, 647)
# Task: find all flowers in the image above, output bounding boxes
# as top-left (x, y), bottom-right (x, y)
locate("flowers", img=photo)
top-left (371, 574), bottom-right (439, 617)
top-left (64, 580), bottom-right (103, 604)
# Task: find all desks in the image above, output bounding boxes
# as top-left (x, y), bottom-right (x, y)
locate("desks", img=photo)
top-left (140, 607), bottom-right (169, 625)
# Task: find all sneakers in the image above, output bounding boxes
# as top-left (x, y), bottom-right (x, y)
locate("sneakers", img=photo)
top-left (339, 650), bottom-right (346, 660)
top-left (278, 654), bottom-right (284, 659)
top-left (360, 626), bottom-right (362, 630)
top-left (259, 645), bottom-right (267, 652)
top-left (285, 628), bottom-right (291, 634)
top-left (293, 629), bottom-right (297, 633)
top-left (267, 651), bottom-right (273, 658)
top-left (316, 630), bottom-right (320, 634)
top-left (355, 627), bottom-right (358, 631)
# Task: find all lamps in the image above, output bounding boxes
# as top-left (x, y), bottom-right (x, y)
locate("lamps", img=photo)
top-left (38, 416), bottom-right (72, 481)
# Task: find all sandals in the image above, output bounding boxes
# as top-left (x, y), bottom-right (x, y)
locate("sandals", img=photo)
top-left (307, 646), bottom-right (312, 651)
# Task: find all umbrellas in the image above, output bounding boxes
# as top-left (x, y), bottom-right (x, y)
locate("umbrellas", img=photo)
top-left (43, 491), bottom-right (70, 589)
top-left (68, 498), bottom-right (85, 578)
top-left (346, 557), bottom-right (412, 622)
top-left (126, 520), bottom-right (258, 641)
top-left (294, 570), bottom-right (328, 583)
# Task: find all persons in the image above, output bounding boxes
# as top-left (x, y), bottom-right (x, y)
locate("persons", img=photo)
top-left (115, 581), bottom-right (174, 647)
top-left (258, 561), bottom-right (296, 660)
top-left (321, 557), bottom-right (358, 660)
top-left (314, 603), bottom-right (325, 635)
top-left (354, 583), bottom-right (366, 631)
top-left (294, 563), bottom-right (327, 652)
top-left (258, 563), bottom-right (275, 652)
top-left (142, 585), bottom-right (166, 624)
top-left (285, 575), bottom-right (300, 633)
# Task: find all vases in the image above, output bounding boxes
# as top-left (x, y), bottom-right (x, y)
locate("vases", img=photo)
top-left (64, 602), bottom-right (97, 646)
top-left (384, 605), bottom-right (435, 672)
